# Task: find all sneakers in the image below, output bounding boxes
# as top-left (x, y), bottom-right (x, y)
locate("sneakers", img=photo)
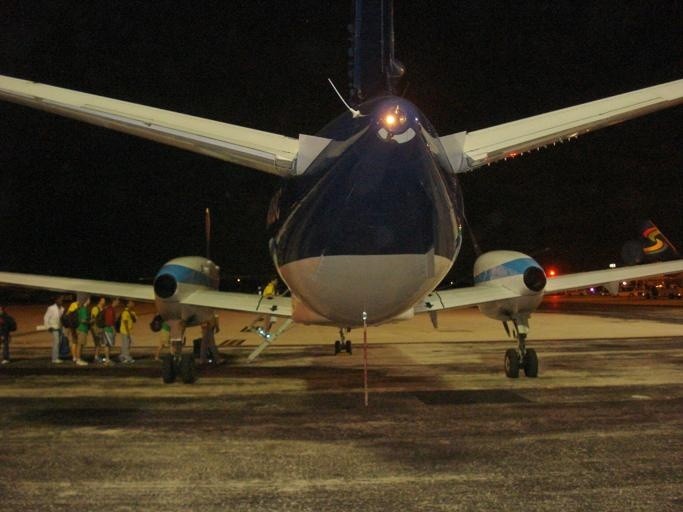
top-left (52, 358), bottom-right (135, 366)
top-left (1, 360), bottom-right (10, 364)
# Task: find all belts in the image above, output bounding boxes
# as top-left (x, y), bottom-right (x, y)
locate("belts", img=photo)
top-left (5, 315), bottom-right (16, 331)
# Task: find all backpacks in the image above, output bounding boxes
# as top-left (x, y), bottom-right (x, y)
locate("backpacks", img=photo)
top-left (60, 310), bottom-right (79, 328)
top-left (150, 315), bottom-right (162, 332)
top-left (96, 306), bottom-right (111, 328)
top-left (114, 309), bottom-right (128, 333)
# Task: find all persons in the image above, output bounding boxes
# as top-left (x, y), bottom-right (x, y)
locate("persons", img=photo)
top-left (261, 276), bottom-right (279, 338)
top-left (0, 307), bottom-right (17, 364)
top-left (43, 294), bottom-right (226, 367)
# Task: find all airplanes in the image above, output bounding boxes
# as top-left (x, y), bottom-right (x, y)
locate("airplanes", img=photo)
top-left (1, 1), bottom-right (683, 383)
